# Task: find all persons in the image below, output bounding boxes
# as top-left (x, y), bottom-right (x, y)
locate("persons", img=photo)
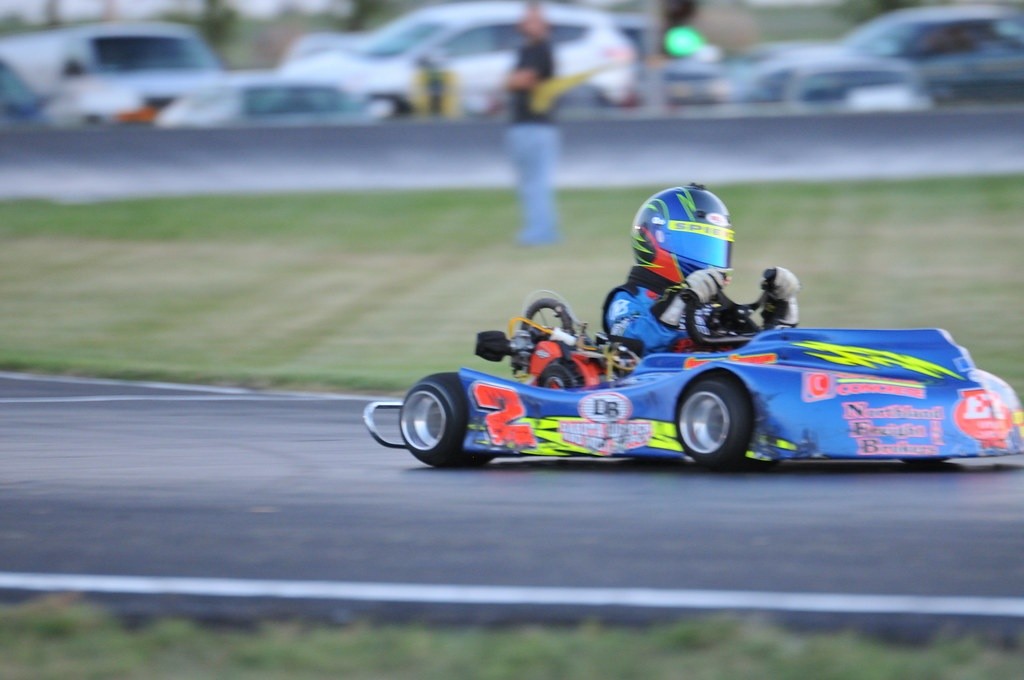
top-left (506, 6), bottom-right (559, 248)
top-left (601, 184), bottom-right (799, 357)
top-left (410, 52), bottom-right (461, 118)
top-left (664, 46), bottom-right (723, 106)
top-left (591, 28), bottom-right (638, 109)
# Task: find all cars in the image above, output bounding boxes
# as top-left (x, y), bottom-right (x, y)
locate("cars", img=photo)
top-left (735, 1), bottom-right (1023, 108)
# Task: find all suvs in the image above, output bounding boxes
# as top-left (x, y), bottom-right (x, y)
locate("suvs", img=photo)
top-left (275, 0), bottom-right (644, 113)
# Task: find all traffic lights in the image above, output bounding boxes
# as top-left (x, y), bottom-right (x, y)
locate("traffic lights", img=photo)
top-left (654, 0), bottom-right (699, 61)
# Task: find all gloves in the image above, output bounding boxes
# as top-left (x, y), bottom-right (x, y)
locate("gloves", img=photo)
top-left (684, 267), bottom-right (734, 304)
top-left (762, 267), bottom-right (801, 302)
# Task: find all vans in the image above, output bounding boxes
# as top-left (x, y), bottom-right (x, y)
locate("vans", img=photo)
top-left (0, 22), bottom-right (246, 130)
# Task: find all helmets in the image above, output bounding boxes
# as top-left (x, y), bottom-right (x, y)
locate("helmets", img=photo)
top-left (629, 180), bottom-right (735, 295)
